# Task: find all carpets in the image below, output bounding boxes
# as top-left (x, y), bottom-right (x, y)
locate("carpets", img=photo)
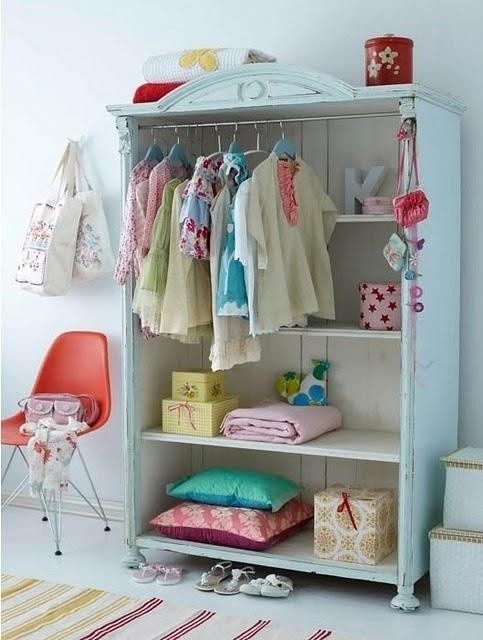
top-left (0, 573), bottom-right (331, 640)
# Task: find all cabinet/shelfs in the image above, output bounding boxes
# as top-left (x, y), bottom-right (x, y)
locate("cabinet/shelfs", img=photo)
top-left (102, 62), bottom-right (468, 614)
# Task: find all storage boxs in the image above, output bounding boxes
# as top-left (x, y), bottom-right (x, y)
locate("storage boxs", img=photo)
top-left (427, 521), bottom-right (483, 614)
top-left (313, 482), bottom-right (398, 565)
top-left (161, 397), bottom-right (239, 437)
top-left (357, 280), bottom-right (402, 332)
top-left (168, 369), bottom-right (225, 403)
top-left (439, 445), bottom-right (483, 531)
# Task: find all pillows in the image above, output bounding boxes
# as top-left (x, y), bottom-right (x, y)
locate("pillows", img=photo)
top-left (149, 499), bottom-right (314, 550)
top-left (163, 465), bottom-right (303, 512)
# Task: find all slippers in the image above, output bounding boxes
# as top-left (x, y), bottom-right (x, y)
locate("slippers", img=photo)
top-left (132, 563), bottom-right (162, 583)
top-left (156, 565), bottom-right (182, 585)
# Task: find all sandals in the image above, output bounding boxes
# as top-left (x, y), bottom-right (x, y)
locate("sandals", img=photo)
top-left (194, 561), bottom-right (232, 592)
top-left (212, 566), bottom-right (255, 594)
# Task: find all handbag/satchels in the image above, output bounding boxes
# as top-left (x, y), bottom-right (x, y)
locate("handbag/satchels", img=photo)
top-left (17, 392), bottom-right (99, 424)
top-left (382, 233), bottom-right (406, 272)
top-left (392, 189), bottom-right (427, 227)
top-left (14, 141), bottom-right (82, 297)
top-left (71, 141), bottom-right (115, 283)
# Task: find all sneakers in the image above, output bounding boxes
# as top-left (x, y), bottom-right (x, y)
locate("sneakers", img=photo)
top-left (239, 578), bottom-right (264, 596)
top-left (260, 573), bottom-right (293, 597)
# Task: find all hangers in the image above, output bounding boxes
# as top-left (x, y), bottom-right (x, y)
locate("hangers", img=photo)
top-left (139, 123), bottom-right (300, 177)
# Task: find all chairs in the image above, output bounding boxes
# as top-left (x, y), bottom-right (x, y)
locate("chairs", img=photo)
top-left (0, 330), bottom-right (112, 556)
